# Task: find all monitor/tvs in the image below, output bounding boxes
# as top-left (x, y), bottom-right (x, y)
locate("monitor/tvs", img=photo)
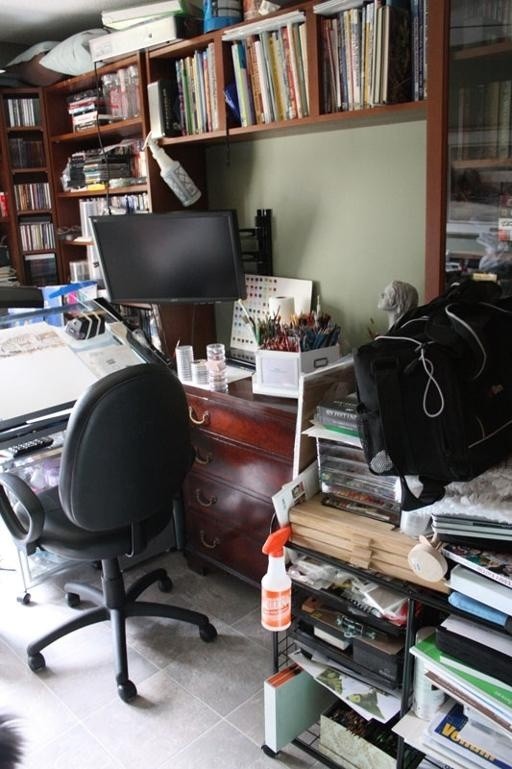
top-left (89, 208), bottom-right (246, 304)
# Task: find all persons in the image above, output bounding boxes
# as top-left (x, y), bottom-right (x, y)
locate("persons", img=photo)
top-left (376, 281), bottom-right (419, 329)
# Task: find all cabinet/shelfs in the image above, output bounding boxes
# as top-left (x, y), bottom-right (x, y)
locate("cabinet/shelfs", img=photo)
top-left (259, 491), bottom-right (512, 769)
top-left (0, 0), bottom-right (512, 304)
top-left (182, 378), bottom-right (297, 591)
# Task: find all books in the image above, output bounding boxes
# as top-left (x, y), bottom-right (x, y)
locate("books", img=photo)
top-left (392, 499), bottom-right (512, 769)
top-left (289, 502), bottom-right (450, 594)
top-left (0, 61), bottom-right (151, 321)
top-left (315, 392), bottom-right (361, 437)
top-left (149, 0), bottom-right (426, 137)
top-left (444, 0), bottom-right (512, 282)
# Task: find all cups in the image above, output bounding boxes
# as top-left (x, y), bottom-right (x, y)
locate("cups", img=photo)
top-left (175, 342), bottom-right (229, 395)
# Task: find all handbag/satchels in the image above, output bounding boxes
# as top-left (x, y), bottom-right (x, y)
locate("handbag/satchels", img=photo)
top-left (352, 274), bottom-right (512, 486)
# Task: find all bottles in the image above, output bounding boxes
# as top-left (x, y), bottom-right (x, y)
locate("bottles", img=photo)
top-left (102, 64), bottom-right (140, 122)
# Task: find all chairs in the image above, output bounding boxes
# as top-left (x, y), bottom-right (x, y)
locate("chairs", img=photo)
top-left (0, 364), bottom-right (218, 703)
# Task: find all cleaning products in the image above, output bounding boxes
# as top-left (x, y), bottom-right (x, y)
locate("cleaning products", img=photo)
top-left (261, 527), bottom-right (293, 633)
top-left (141, 134), bottom-right (201, 207)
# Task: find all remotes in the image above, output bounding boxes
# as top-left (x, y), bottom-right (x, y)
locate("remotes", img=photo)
top-left (7, 435), bottom-right (54, 457)
